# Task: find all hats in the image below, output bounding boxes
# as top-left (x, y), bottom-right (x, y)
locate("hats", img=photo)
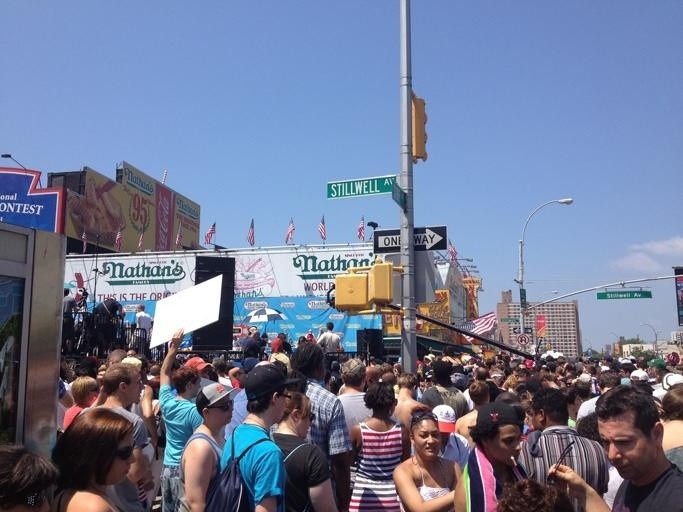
top-left (662, 372), bottom-right (683, 389)
top-left (196, 382), bottom-right (240, 414)
top-left (630, 370), bottom-right (648, 381)
top-left (433, 404), bottom-right (456, 434)
top-left (476, 403), bottom-right (519, 427)
top-left (244, 362), bottom-right (300, 401)
top-left (647, 359), bottom-right (666, 368)
top-left (184, 357), bottom-right (213, 372)
top-left (423, 353), bottom-right (435, 362)
top-left (451, 373), bottom-right (469, 388)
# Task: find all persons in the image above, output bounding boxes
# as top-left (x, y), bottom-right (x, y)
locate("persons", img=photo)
top-left (62, 288), bottom-right (154, 355)
top-left (1, 443), bottom-right (61, 511)
top-left (51, 323), bottom-right (682, 512)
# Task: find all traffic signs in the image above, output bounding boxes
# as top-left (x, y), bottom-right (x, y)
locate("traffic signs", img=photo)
top-left (499, 317), bottom-right (520, 323)
top-left (512, 328), bottom-right (531, 333)
top-left (519, 289), bottom-right (526, 308)
top-left (326, 174), bottom-right (396, 200)
top-left (392, 181), bottom-right (406, 210)
top-left (596, 290), bottom-right (652, 300)
top-left (372, 225), bottom-right (448, 253)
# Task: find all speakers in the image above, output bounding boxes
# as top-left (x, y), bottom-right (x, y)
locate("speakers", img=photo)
top-left (356, 328), bottom-right (385, 367)
top-left (192, 255), bottom-right (236, 350)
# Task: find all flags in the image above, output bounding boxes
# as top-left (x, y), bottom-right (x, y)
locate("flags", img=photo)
top-left (82, 233), bottom-right (88, 253)
top-left (449, 241), bottom-right (457, 258)
top-left (204, 222), bottom-right (216, 245)
top-left (286, 218), bottom-right (295, 243)
top-left (317, 216), bottom-right (326, 240)
top-left (115, 225), bottom-right (123, 252)
top-left (138, 228), bottom-right (144, 251)
top-left (247, 218), bottom-right (255, 246)
top-left (357, 216), bottom-right (364, 240)
top-left (176, 222), bottom-right (184, 249)
top-left (459, 312), bottom-right (500, 343)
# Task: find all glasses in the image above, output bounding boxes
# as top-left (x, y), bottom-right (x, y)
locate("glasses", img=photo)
top-left (115, 442), bottom-right (135, 459)
top-left (209, 400), bottom-right (234, 411)
top-left (412, 411), bottom-right (439, 422)
top-left (19, 494), bottom-right (44, 508)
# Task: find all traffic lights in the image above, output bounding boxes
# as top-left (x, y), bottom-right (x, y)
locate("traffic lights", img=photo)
top-left (412, 100), bottom-right (427, 160)
top-left (368, 261), bottom-right (393, 303)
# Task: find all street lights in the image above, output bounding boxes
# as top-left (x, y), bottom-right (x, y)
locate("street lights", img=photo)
top-left (639, 323), bottom-right (662, 345)
top-left (533, 290), bottom-right (559, 347)
top-left (517, 197), bottom-right (573, 363)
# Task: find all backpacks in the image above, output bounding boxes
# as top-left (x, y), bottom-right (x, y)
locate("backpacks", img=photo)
top-left (206, 427), bottom-right (271, 512)
